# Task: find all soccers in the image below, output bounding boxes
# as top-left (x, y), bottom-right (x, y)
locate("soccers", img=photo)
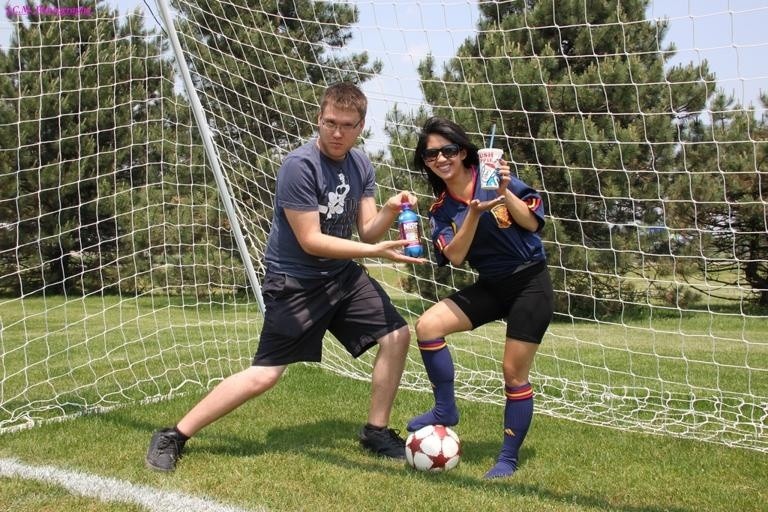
top-left (405, 425), bottom-right (461, 473)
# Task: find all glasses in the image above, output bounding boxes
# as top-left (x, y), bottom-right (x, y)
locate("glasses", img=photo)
top-left (320, 117), bottom-right (363, 131)
top-left (422, 144), bottom-right (463, 162)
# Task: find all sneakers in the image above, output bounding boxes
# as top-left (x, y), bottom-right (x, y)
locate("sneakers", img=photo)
top-left (359, 426), bottom-right (405, 461)
top-left (144, 429), bottom-right (185, 471)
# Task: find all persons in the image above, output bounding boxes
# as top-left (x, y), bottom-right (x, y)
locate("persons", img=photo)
top-left (406, 117), bottom-right (555, 480)
top-left (146, 81), bottom-right (427, 472)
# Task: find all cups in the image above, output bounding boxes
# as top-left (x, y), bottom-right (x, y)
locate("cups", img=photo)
top-left (479, 149), bottom-right (503, 190)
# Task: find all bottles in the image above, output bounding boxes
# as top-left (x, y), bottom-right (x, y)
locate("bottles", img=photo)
top-left (399, 202), bottom-right (423, 257)
